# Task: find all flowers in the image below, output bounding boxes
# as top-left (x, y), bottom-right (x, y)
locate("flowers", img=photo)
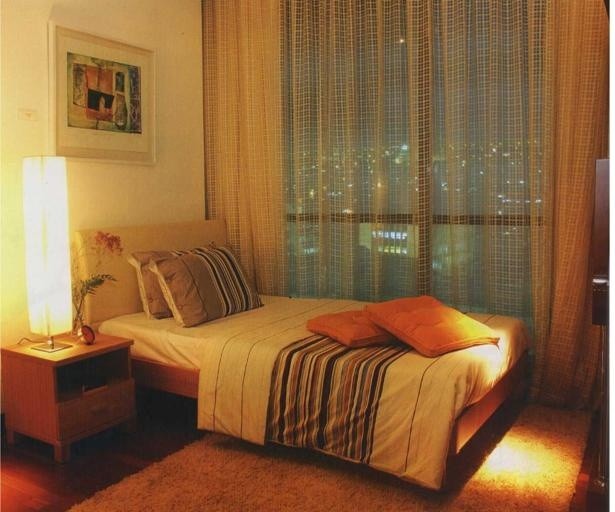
top-left (69, 230), bottom-right (123, 330)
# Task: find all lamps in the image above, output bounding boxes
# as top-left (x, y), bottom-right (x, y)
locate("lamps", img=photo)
top-left (20, 155), bottom-right (76, 353)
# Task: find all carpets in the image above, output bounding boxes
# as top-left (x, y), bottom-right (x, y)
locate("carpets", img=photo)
top-left (58, 396), bottom-right (595, 511)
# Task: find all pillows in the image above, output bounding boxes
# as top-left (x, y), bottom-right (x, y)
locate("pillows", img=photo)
top-left (306, 295), bottom-right (501, 358)
top-left (126, 241), bottom-right (265, 328)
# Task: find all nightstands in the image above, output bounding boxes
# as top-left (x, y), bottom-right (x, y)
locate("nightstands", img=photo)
top-left (1, 327), bottom-right (136, 467)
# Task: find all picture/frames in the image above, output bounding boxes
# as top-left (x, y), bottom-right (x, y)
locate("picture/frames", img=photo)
top-left (47, 21), bottom-right (157, 167)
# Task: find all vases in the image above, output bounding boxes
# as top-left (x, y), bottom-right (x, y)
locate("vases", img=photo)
top-left (72, 296), bottom-right (85, 337)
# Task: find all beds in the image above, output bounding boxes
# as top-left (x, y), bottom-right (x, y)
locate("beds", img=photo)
top-left (75, 218), bottom-right (528, 491)
